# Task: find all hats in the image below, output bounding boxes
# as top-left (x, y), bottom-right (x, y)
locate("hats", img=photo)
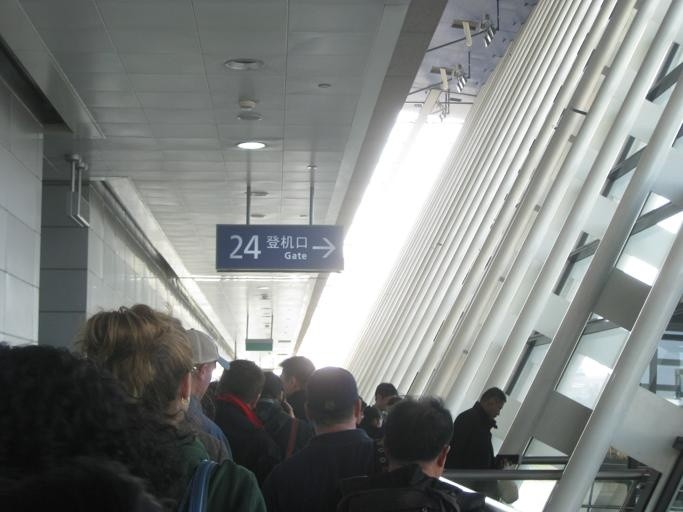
top-left (306, 367), bottom-right (361, 413)
top-left (186, 330), bottom-right (231, 371)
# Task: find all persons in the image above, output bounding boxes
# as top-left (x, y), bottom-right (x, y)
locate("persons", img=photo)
top-left (1, 339), bottom-right (173, 512)
top-left (178, 326), bottom-right (236, 464)
top-left (66, 301), bottom-right (267, 512)
top-left (200, 357), bottom-right (509, 512)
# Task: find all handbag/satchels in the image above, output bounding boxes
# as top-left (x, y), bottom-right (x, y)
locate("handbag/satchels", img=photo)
top-left (497, 478), bottom-right (518, 502)
